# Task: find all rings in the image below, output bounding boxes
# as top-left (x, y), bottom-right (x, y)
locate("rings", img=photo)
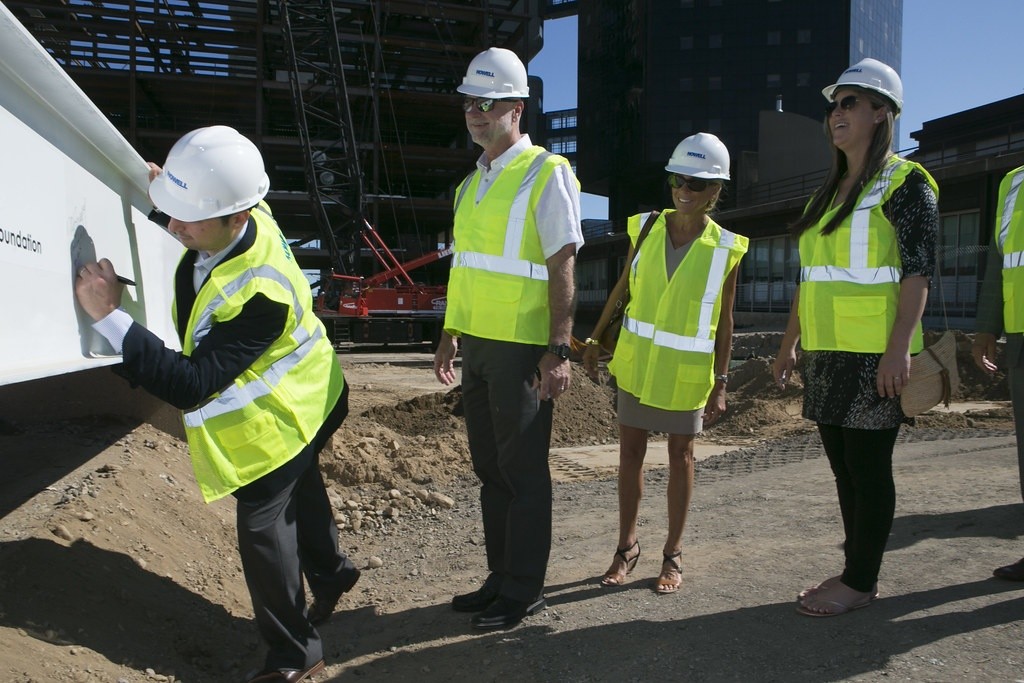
top-left (80, 267), bottom-right (88, 276)
top-left (894, 376), bottom-right (900, 379)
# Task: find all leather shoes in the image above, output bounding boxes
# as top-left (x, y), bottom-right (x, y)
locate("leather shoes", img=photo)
top-left (453, 573), bottom-right (501, 611)
top-left (470, 596), bottom-right (547, 630)
top-left (307, 567), bottom-right (361, 626)
top-left (246, 657), bottom-right (325, 683)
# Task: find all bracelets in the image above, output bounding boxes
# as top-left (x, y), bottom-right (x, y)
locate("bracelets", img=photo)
top-left (714, 374), bottom-right (728, 384)
top-left (586, 338), bottom-right (601, 346)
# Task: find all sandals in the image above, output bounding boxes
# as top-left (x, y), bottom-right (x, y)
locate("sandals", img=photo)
top-left (601, 539), bottom-right (640, 586)
top-left (797, 576), bottom-right (842, 601)
top-left (656, 547), bottom-right (682, 593)
top-left (795, 581), bottom-right (880, 616)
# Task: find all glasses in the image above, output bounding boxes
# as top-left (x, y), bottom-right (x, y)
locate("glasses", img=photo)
top-left (826, 96), bottom-right (860, 117)
top-left (668, 174), bottom-right (706, 192)
top-left (463, 96), bottom-right (518, 113)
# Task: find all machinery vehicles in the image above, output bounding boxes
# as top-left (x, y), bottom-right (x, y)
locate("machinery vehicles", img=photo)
top-left (276, 0), bottom-right (462, 351)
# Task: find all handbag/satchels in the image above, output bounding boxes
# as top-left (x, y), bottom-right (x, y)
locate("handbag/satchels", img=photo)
top-left (899, 331), bottom-right (958, 418)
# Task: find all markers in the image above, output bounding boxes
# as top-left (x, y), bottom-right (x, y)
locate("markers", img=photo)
top-left (534, 367), bottom-right (550, 398)
top-left (118, 277), bottom-right (137, 286)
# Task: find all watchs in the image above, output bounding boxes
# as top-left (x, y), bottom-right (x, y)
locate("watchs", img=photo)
top-left (547, 342), bottom-right (571, 360)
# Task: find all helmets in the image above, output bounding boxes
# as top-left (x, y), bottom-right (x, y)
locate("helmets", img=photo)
top-left (457, 47), bottom-right (530, 99)
top-left (665, 132), bottom-right (730, 180)
top-left (149, 125), bottom-right (269, 222)
top-left (822, 59), bottom-right (903, 110)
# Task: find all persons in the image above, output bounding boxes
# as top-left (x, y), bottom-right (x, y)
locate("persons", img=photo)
top-left (772, 57), bottom-right (939, 616)
top-left (75, 124), bottom-right (360, 683)
top-left (969, 165), bottom-right (1024, 585)
top-left (579, 132), bottom-right (750, 594)
top-left (434, 47), bottom-right (586, 629)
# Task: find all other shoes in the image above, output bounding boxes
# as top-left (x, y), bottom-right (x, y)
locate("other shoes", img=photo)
top-left (993, 558), bottom-right (1023, 580)
